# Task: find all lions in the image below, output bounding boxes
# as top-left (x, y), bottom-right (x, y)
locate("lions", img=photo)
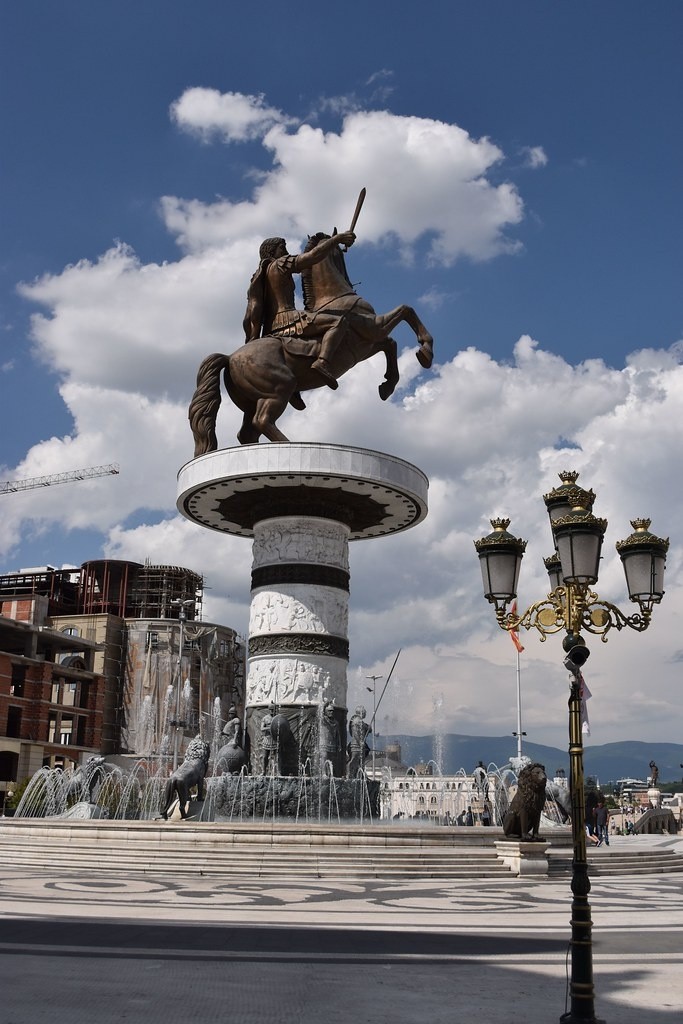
top-left (512, 755), bottom-right (573, 826)
top-left (501, 762), bottom-right (549, 843)
top-left (53, 755), bottom-right (107, 816)
top-left (157, 731), bottom-right (212, 821)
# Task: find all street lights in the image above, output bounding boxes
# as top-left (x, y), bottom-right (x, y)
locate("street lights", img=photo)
top-left (472, 469), bottom-right (672, 1024)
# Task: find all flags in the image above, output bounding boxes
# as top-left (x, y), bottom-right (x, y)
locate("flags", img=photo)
top-left (507, 600), bottom-right (525, 653)
top-left (578, 670), bottom-right (593, 737)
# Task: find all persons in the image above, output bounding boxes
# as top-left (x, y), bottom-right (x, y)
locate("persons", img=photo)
top-left (222, 706), bottom-right (241, 749)
top-left (317, 704), bottom-right (342, 776)
top-left (625, 819), bottom-right (634, 835)
top-left (586, 800), bottom-right (610, 847)
top-left (259, 230), bottom-right (357, 411)
top-left (444, 811), bottom-right (455, 826)
top-left (482, 805), bottom-right (491, 826)
top-left (349, 706), bottom-right (372, 780)
top-left (649, 761), bottom-right (659, 785)
top-left (259, 700), bottom-right (280, 777)
top-left (610, 817), bottom-right (615, 835)
top-left (457, 806), bottom-right (477, 826)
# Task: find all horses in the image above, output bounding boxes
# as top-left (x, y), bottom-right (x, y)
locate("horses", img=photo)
top-left (186, 225), bottom-right (436, 459)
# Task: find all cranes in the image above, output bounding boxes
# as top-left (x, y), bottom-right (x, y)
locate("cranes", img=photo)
top-left (0, 461), bottom-right (120, 495)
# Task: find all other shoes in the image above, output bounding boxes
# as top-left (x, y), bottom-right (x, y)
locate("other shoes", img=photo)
top-left (595, 841), bottom-right (601, 847)
top-left (606, 841), bottom-right (609, 845)
